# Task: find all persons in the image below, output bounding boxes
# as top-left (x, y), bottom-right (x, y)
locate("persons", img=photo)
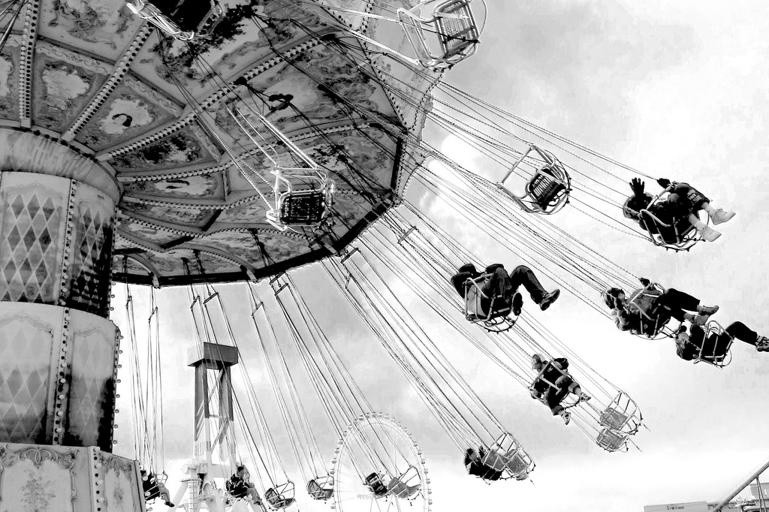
top-left (622, 177), bottom-right (736, 241)
top-left (450, 263), bottom-right (559, 320)
top-left (227, 467), bottom-right (261, 504)
top-left (465, 446), bottom-right (502, 480)
top-left (529, 354), bottom-right (590, 425)
top-left (140, 469), bottom-right (174, 506)
top-left (604, 278), bottom-right (720, 336)
top-left (675, 324), bottom-right (768, 362)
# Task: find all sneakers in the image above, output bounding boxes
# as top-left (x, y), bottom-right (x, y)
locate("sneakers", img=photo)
top-left (580, 393), bottom-right (591, 402)
top-left (712, 208), bottom-right (736, 225)
top-left (540, 289), bottom-right (559, 311)
top-left (699, 305), bottom-right (719, 317)
top-left (561, 411), bottom-right (571, 425)
top-left (692, 315), bottom-right (709, 326)
top-left (756, 336), bottom-right (769, 352)
top-left (512, 292), bottom-right (523, 316)
top-left (700, 226), bottom-right (722, 243)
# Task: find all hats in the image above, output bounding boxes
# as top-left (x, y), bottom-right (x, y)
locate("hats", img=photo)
top-left (676, 326), bottom-right (687, 343)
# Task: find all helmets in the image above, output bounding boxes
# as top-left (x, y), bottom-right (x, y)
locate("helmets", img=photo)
top-left (623, 196), bottom-right (647, 220)
top-left (604, 287), bottom-right (624, 309)
top-left (530, 353), bottom-right (546, 369)
